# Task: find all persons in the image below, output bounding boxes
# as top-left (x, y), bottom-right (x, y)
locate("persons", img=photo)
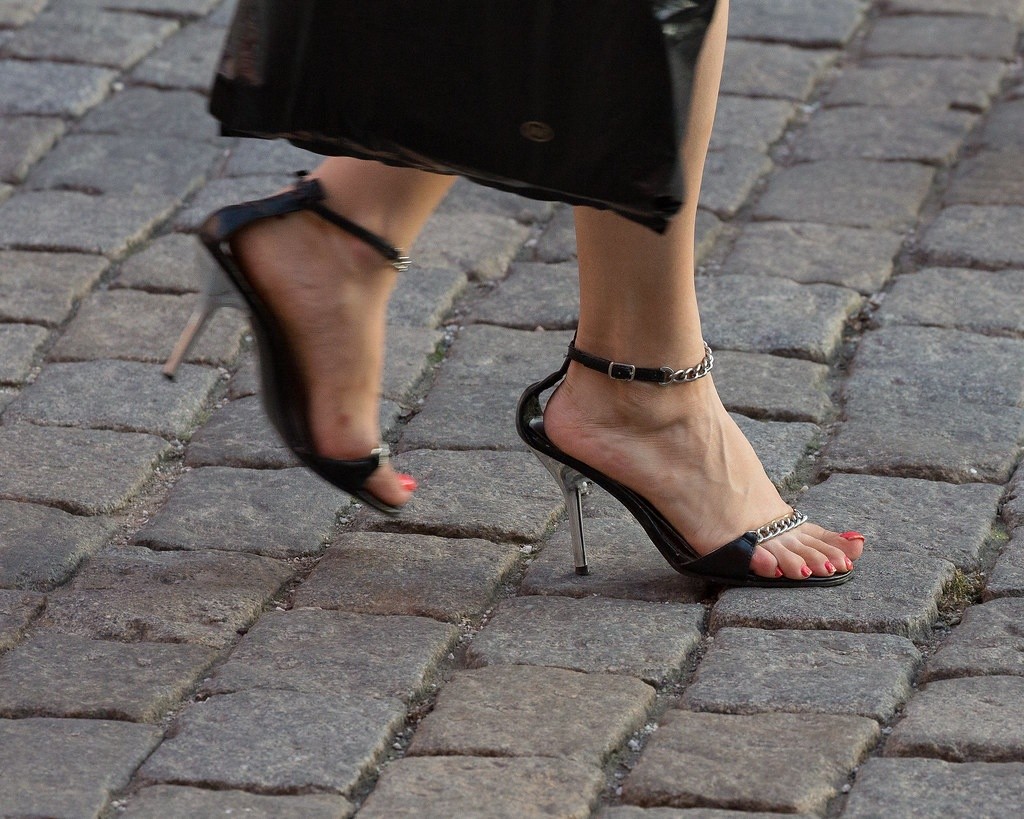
top-left (164, 0), bottom-right (863, 589)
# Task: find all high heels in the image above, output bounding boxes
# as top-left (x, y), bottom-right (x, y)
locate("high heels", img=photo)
top-left (162, 168), bottom-right (417, 518)
top-left (515, 328), bottom-right (866, 589)
